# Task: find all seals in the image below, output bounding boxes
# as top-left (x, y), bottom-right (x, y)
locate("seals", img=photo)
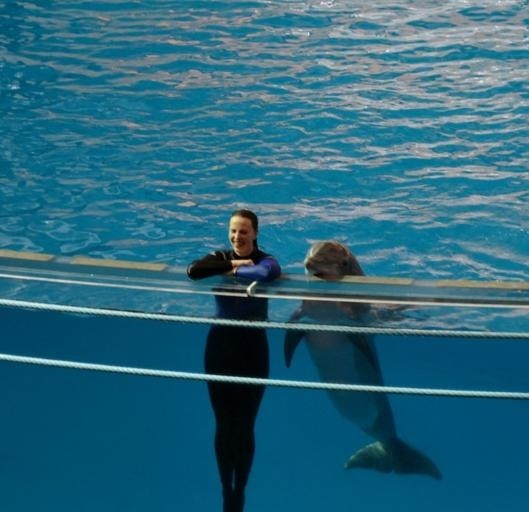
top-left (286, 236), bottom-right (444, 483)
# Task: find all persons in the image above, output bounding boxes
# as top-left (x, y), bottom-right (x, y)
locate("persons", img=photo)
top-left (186, 210), bottom-right (280, 512)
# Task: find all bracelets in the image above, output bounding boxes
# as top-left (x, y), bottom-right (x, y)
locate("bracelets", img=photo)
top-left (233, 266), bottom-right (238, 277)
